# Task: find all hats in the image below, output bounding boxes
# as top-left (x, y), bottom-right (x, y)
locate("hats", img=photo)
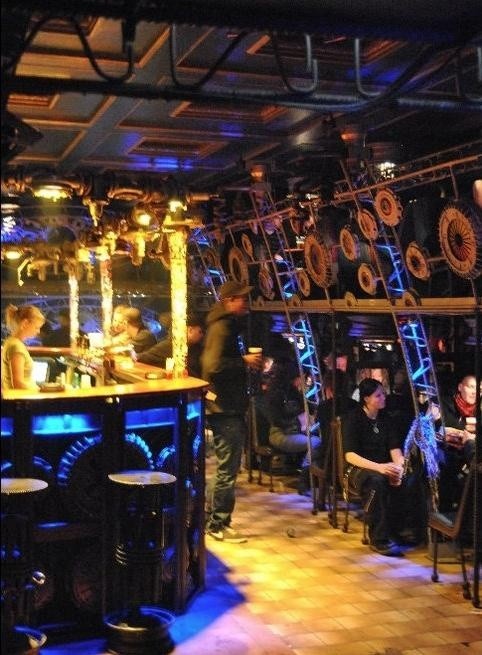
top-left (218, 280), bottom-right (255, 299)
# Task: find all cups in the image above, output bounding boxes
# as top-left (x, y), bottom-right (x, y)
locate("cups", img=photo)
top-left (248, 347), bottom-right (261, 375)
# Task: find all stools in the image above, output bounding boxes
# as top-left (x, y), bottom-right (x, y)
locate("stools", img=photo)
top-left (106, 473), bottom-right (181, 652)
top-left (0, 476), bottom-right (51, 655)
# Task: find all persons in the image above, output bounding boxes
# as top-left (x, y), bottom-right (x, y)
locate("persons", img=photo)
top-left (112, 309), bottom-right (164, 370)
top-left (140, 313), bottom-right (207, 379)
top-left (0, 303), bottom-right (46, 389)
top-left (198, 281), bottom-right (255, 544)
top-left (43, 308), bottom-right (87, 372)
top-left (105, 304), bottom-right (131, 346)
top-left (341, 376), bottom-right (424, 557)
top-left (434, 368), bottom-right (482, 438)
top-left (248, 352), bottom-right (274, 397)
top-left (312, 369), bottom-right (359, 512)
top-left (264, 362), bottom-right (321, 495)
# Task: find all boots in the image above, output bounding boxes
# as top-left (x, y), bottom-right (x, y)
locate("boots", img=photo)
top-left (299, 466), bottom-right (311, 497)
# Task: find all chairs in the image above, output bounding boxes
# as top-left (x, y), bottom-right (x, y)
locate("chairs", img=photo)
top-left (246, 398), bottom-right (284, 490)
top-left (424, 455), bottom-right (478, 597)
top-left (330, 417), bottom-right (386, 544)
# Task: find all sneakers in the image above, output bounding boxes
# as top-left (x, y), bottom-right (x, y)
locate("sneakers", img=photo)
top-left (208, 525), bottom-right (248, 544)
top-left (370, 539), bottom-right (401, 555)
